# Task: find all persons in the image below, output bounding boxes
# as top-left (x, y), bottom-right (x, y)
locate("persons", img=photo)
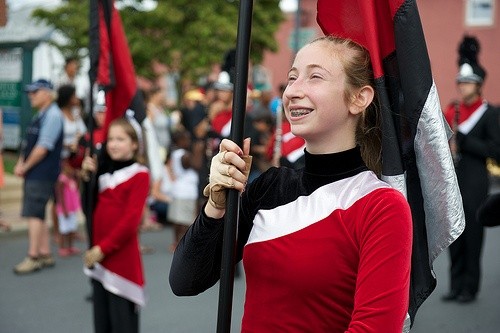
top-left (80, 119), bottom-right (152, 333)
top-left (13, 79), bottom-right (66, 276)
top-left (50, 33), bottom-right (305, 299)
top-left (442, 34), bottom-right (500, 302)
top-left (168, 37), bottom-right (413, 333)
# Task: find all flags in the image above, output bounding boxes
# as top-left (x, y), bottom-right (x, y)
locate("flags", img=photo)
top-left (91, 0), bottom-right (137, 142)
top-left (316, 0), bottom-right (468, 330)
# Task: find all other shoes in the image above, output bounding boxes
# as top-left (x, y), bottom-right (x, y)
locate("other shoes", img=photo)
top-left (14, 255), bottom-right (43, 274)
top-left (40, 254), bottom-right (55, 266)
top-left (442, 290), bottom-right (457, 300)
top-left (457, 289), bottom-right (475, 302)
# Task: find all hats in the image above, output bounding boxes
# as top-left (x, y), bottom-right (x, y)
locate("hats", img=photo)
top-left (214, 50), bottom-right (236, 91)
top-left (455, 35), bottom-right (486, 84)
top-left (23, 79), bottom-right (54, 92)
